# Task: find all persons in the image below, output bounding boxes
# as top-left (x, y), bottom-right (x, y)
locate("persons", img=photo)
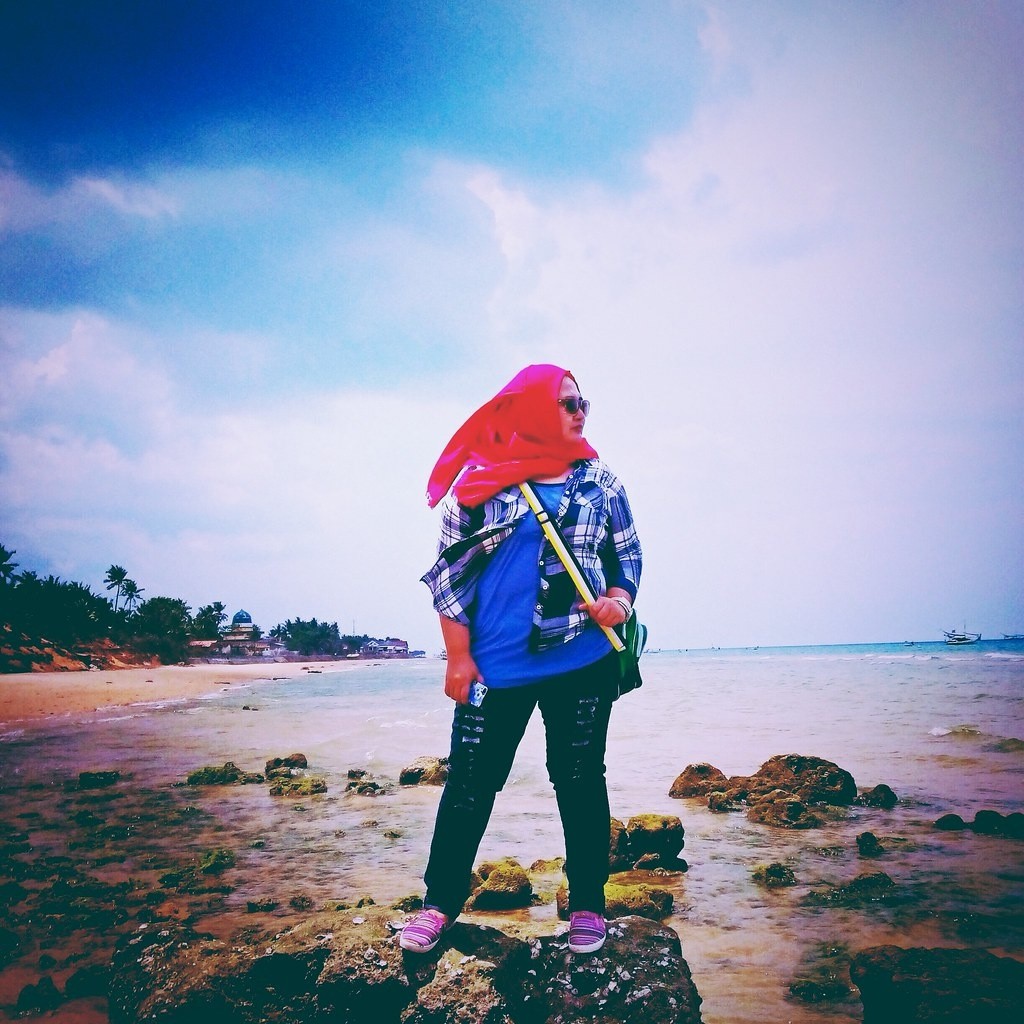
top-left (400, 363), bottom-right (643, 957)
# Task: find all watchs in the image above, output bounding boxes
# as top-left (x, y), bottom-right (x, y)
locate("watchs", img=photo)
top-left (612, 596), bottom-right (633, 624)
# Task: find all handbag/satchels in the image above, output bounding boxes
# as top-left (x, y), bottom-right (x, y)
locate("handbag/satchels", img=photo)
top-left (611, 608), bottom-right (647, 701)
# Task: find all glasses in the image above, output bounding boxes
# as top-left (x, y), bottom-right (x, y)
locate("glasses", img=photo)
top-left (558, 398), bottom-right (591, 415)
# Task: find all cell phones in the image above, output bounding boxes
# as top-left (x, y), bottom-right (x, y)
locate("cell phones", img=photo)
top-left (468, 679), bottom-right (488, 708)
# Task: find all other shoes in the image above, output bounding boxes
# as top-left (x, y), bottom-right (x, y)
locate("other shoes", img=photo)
top-left (400, 910), bottom-right (458, 954)
top-left (568, 912), bottom-right (607, 954)
